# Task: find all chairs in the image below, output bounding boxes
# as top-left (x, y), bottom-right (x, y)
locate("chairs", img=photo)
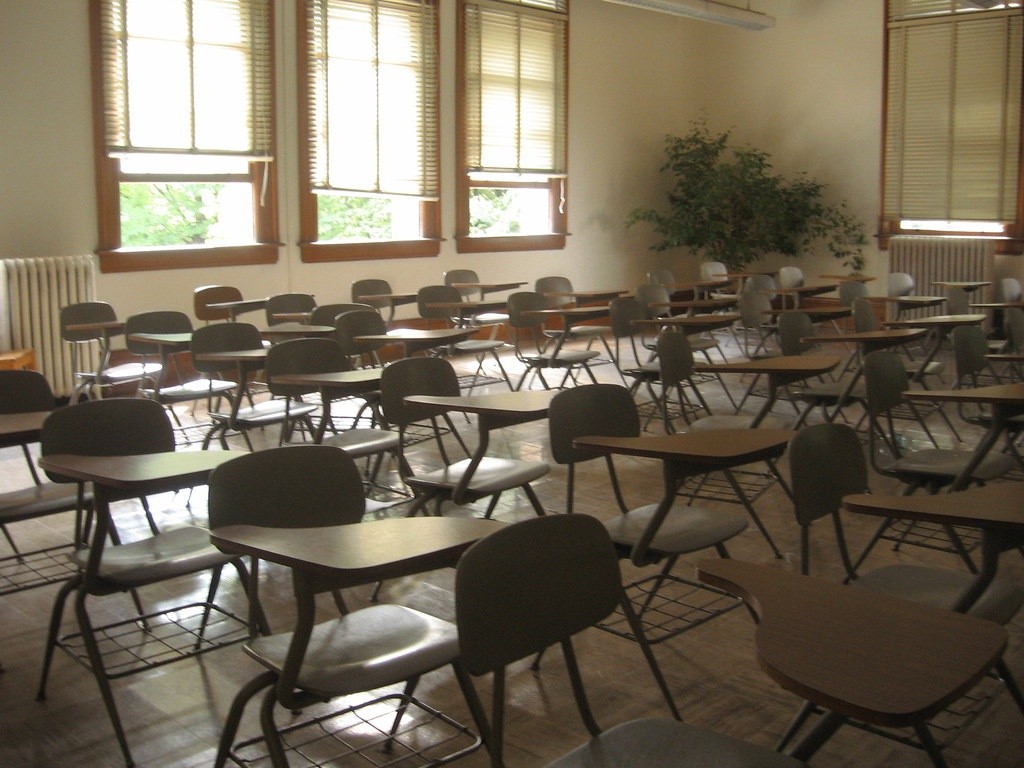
top-left (0, 260), bottom-right (1024, 768)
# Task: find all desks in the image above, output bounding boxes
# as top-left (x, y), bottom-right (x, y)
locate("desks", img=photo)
top-left (401, 389), bottom-right (560, 506)
top-left (627, 314), bottom-right (744, 339)
top-left (900, 383), bottom-right (1024, 492)
top-left (205, 293), bottom-right (316, 323)
top-left (520, 305), bottom-right (611, 368)
top-left (424, 299), bottom-right (507, 353)
top-left (351, 326), bottom-right (483, 358)
top-left (0, 411), bottom-right (53, 449)
top-left (38, 445), bottom-right (250, 594)
top-left (839, 481), bottom-right (1024, 614)
top-left (358, 293), bottom-right (418, 326)
top-left (208, 516), bottom-right (512, 709)
top-left (574, 428), bottom-right (798, 567)
top-left (692, 557), bottom-right (1024, 768)
top-left (129, 332), bottom-right (193, 401)
top-left (66, 321), bottom-right (126, 384)
top-left (195, 349), bottom-right (269, 430)
top-left (257, 321), bottom-right (338, 346)
top-left (692, 354), bottom-right (844, 425)
top-left (271, 367), bottom-right (384, 445)
top-left (273, 312), bottom-right (314, 321)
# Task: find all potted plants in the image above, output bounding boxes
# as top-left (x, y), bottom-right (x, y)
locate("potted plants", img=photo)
top-left (618, 106), bottom-right (869, 288)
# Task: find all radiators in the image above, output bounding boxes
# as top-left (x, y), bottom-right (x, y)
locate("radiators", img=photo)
top-left (0, 254), bottom-right (104, 400)
top-left (885, 234), bottom-right (996, 335)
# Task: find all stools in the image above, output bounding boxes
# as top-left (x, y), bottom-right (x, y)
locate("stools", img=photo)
top-left (0, 349), bottom-right (35, 370)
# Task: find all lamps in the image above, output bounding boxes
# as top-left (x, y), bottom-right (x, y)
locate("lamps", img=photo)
top-left (606, 0), bottom-right (776, 33)
